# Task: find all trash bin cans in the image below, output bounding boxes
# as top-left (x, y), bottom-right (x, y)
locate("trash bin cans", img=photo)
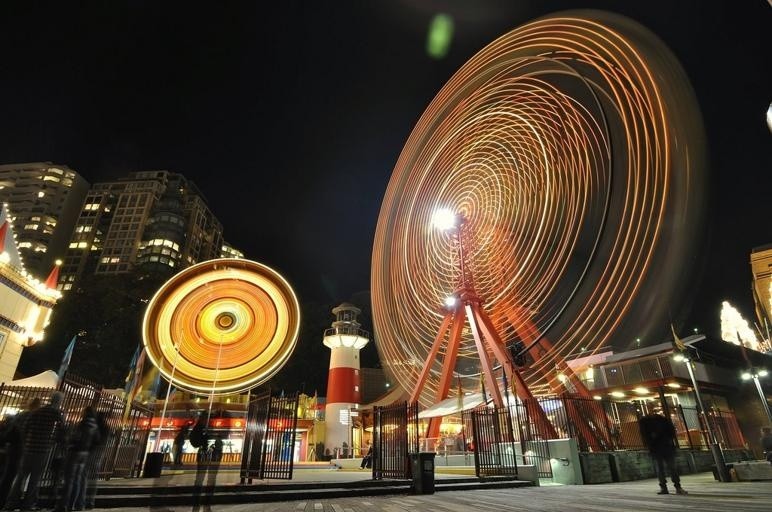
top-left (408, 452), bottom-right (436, 495)
top-left (142, 452), bottom-right (164, 478)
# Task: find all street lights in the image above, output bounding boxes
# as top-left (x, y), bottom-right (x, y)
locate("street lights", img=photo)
top-left (673, 353), bottom-right (729, 482)
top-left (739, 367), bottom-right (771, 427)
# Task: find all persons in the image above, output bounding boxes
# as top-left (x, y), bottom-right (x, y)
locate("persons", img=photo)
top-left (191, 396), bottom-right (232, 511)
top-left (173, 425), bottom-right (189, 465)
top-left (359, 446), bottom-right (372, 469)
top-left (0, 391), bottom-right (109, 512)
top-left (342, 441), bottom-right (348, 457)
top-left (454, 427), bottom-right (467, 451)
top-left (760, 427), bottom-right (771, 465)
top-left (639, 402), bottom-right (688, 495)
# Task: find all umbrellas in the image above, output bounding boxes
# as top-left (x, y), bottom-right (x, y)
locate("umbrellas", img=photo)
top-left (502, 366), bottom-right (509, 396)
top-left (510, 367), bottom-right (518, 399)
top-left (456, 375), bottom-right (464, 409)
top-left (480, 371), bottom-right (488, 403)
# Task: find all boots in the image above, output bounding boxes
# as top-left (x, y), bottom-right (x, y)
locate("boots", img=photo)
top-left (675, 482), bottom-right (688, 494)
top-left (656, 484), bottom-right (670, 495)
